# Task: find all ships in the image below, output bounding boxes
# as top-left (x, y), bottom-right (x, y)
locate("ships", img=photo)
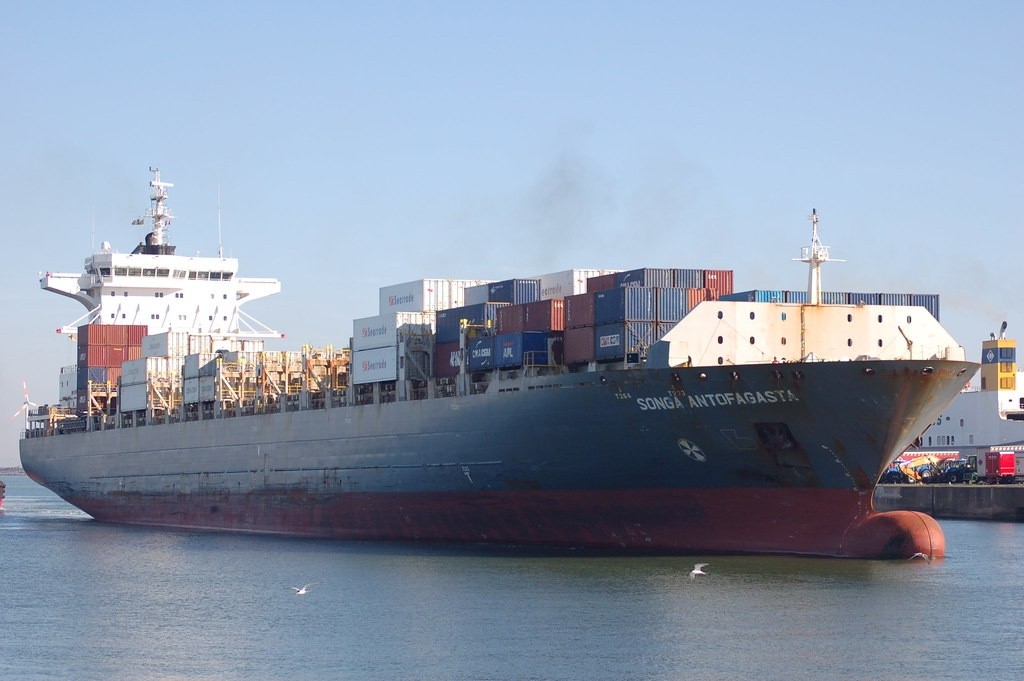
top-left (13, 164), bottom-right (982, 560)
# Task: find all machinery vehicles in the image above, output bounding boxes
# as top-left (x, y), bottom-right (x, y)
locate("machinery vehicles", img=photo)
top-left (878, 454), bottom-right (979, 484)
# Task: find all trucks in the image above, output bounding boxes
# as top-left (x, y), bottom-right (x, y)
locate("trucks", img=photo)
top-left (985, 451), bottom-right (1015, 484)
